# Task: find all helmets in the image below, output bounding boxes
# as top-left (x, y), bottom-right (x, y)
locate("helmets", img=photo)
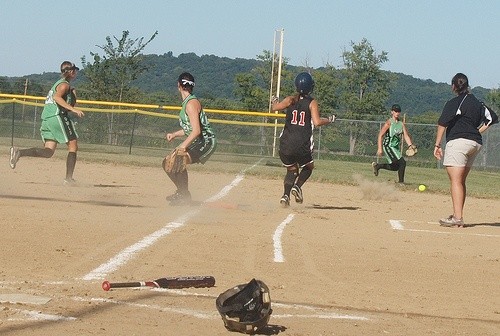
top-left (216, 277), bottom-right (273, 336)
top-left (294, 71), bottom-right (314, 90)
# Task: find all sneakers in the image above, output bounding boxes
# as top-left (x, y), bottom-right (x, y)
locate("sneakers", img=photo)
top-left (398, 180), bottom-right (407, 187)
top-left (63, 176), bottom-right (78, 186)
top-left (166, 190), bottom-right (192, 206)
top-left (280, 194), bottom-right (290, 208)
top-left (371, 161), bottom-right (379, 177)
top-left (9, 147), bottom-right (19, 169)
top-left (439, 215), bottom-right (465, 228)
top-left (290, 184), bottom-right (303, 204)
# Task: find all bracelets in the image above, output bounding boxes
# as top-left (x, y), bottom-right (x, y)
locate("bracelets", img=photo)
top-left (434, 145), bottom-right (440, 148)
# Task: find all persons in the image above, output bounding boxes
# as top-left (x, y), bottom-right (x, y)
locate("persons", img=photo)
top-left (162, 72), bottom-right (217, 205)
top-left (372, 104), bottom-right (417, 187)
top-left (8, 60), bottom-right (85, 185)
top-left (433, 72), bottom-right (499, 226)
top-left (271, 72), bottom-right (337, 206)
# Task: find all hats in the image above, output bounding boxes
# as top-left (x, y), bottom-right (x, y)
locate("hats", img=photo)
top-left (390, 104), bottom-right (401, 113)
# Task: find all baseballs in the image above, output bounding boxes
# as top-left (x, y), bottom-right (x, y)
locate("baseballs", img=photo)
top-left (419, 185), bottom-right (426, 191)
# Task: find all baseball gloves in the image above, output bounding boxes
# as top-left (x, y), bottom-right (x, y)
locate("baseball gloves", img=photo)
top-left (405, 145), bottom-right (418, 156)
top-left (164, 147), bottom-right (188, 174)
top-left (72, 88), bottom-right (78, 106)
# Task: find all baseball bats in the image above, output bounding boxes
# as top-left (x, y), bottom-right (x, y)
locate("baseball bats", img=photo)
top-left (102, 275), bottom-right (215, 291)
